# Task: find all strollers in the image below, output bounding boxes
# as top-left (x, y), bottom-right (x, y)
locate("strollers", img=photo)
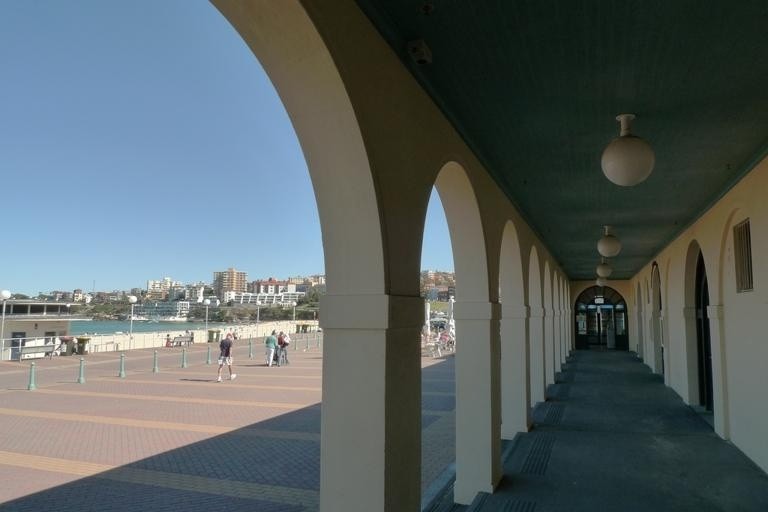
top-left (267, 346), bottom-right (280, 366)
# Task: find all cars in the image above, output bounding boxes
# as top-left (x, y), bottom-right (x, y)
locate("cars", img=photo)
top-left (430, 318), bottom-right (447, 330)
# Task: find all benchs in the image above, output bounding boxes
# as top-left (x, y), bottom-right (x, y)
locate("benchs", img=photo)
top-left (15, 345), bottom-right (55, 363)
top-left (166, 336), bottom-right (191, 346)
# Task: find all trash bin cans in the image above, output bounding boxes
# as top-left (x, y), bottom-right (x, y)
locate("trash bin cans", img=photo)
top-left (75, 337), bottom-right (91, 355)
top-left (296, 324), bottom-right (309, 333)
top-left (216, 330), bottom-right (224, 342)
top-left (208, 329), bottom-right (216, 342)
top-left (59, 336), bottom-right (74, 356)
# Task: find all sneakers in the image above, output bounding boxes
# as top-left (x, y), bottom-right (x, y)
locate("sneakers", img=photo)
top-left (229, 373), bottom-right (236, 380)
top-left (218, 377), bottom-right (222, 383)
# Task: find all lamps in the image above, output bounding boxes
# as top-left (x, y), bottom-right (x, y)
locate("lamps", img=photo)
top-left (596, 256), bottom-right (612, 277)
top-left (595, 222), bottom-right (623, 257)
top-left (594, 276), bottom-right (608, 286)
top-left (600, 113), bottom-right (656, 187)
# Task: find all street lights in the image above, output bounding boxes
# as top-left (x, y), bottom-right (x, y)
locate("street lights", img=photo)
top-left (203, 297), bottom-right (212, 343)
top-left (128, 296), bottom-right (138, 350)
top-left (292, 301), bottom-right (297, 320)
top-left (0, 289), bottom-right (12, 339)
top-left (255, 300), bottom-right (261, 337)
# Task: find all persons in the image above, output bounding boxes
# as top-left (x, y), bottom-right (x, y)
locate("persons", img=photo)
top-left (264, 326), bottom-right (290, 367)
top-left (162, 328), bottom-right (191, 346)
top-left (428, 324), bottom-right (455, 359)
top-left (216, 333), bottom-right (236, 382)
